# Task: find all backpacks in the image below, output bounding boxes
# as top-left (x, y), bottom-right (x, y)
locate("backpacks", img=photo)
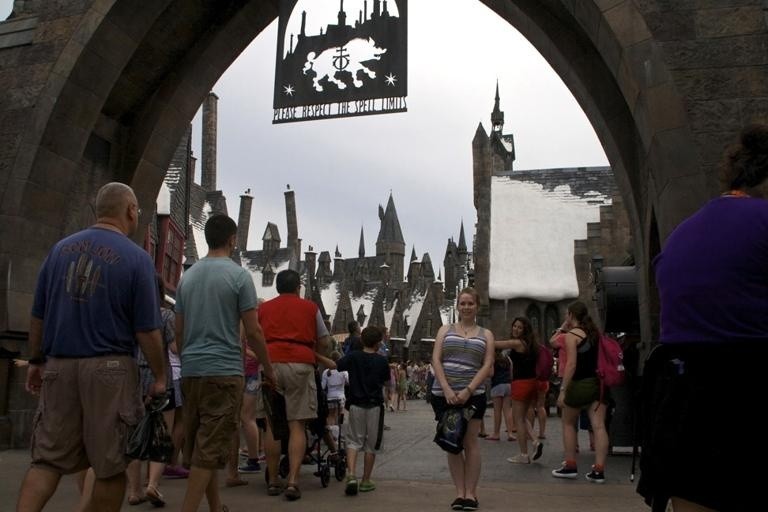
top-left (596, 334), bottom-right (625, 386)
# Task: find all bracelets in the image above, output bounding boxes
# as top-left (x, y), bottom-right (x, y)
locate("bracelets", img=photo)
top-left (27, 355), bottom-right (41, 364)
top-left (466, 386), bottom-right (474, 394)
top-left (560, 385), bottom-right (566, 391)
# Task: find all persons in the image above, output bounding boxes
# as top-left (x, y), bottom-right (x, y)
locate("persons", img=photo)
top-left (479, 330), bottom-right (558, 464)
top-left (657, 120), bottom-right (767, 511)
top-left (384, 358), bottom-right (433, 412)
top-left (15, 182), bottom-right (391, 511)
top-left (549, 308), bottom-right (599, 452)
top-left (432, 285), bottom-right (494, 510)
top-left (551, 300), bottom-right (609, 483)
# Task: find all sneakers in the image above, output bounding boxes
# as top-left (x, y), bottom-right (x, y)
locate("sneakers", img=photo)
top-left (463, 496), bottom-right (480, 510)
top-left (238, 463), bottom-right (262, 474)
top-left (477, 429), bottom-right (605, 481)
top-left (451, 498), bottom-right (463, 510)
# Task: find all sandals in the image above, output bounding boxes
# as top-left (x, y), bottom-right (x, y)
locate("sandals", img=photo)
top-left (128, 460), bottom-right (377, 512)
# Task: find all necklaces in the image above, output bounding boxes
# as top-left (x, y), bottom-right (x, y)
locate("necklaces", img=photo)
top-left (460, 321), bottom-right (475, 336)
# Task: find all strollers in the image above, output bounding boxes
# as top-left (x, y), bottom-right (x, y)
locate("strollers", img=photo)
top-left (267, 381), bottom-right (349, 489)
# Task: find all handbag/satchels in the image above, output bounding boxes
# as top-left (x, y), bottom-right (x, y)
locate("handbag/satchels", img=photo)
top-left (535, 342), bottom-right (554, 381)
